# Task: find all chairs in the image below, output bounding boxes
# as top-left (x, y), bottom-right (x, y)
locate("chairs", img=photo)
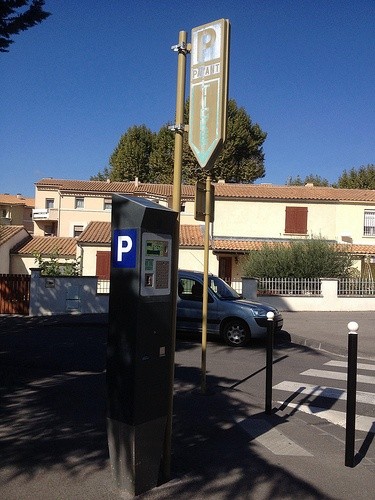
top-left (191, 285), bottom-right (202, 299)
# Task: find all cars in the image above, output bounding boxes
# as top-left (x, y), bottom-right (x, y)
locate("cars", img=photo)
top-left (176, 269), bottom-right (284, 347)
